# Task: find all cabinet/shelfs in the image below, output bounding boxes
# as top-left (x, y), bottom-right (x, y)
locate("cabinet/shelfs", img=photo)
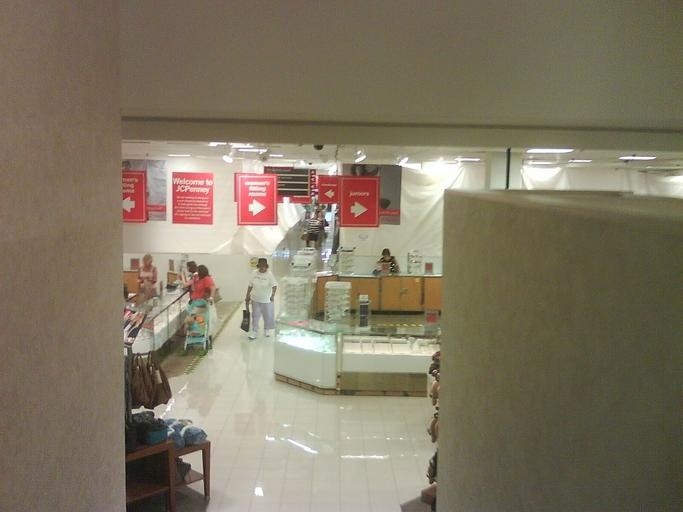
top-left (379, 277), bottom-right (422, 313)
top-left (317, 275), bottom-right (338, 314)
top-left (124, 435), bottom-right (210, 512)
top-left (339, 277), bottom-right (379, 314)
top-left (422, 277), bottom-right (443, 312)
top-left (121, 287), bottom-right (191, 356)
top-left (273, 315), bottom-right (343, 397)
top-left (341, 331), bottom-right (439, 397)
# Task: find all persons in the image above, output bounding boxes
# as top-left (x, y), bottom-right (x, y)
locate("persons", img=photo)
top-left (180, 260), bottom-right (198, 287)
top-left (189, 263), bottom-right (217, 306)
top-left (135, 253), bottom-right (159, 299)
top-left (244, 257), bottom-right (278, 341)
top-left (376, 246), bottom-right (401, 274)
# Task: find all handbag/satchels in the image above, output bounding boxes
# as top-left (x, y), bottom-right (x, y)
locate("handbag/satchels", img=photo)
top-left (204, 288), bottom-right (223, 305)
top-left (241, 300), bottom-right (250, 332)
top-left (130, 351), bottom-right (172, 409)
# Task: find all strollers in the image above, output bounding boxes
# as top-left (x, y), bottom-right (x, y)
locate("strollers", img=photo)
top-left (178, 299), bottom-right (212, 358)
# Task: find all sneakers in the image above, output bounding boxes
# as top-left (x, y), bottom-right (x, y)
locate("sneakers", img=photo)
top-left (265, 329), bottom-right (270, 337)
top-left (248, 331), bottom-right (257, 339)
